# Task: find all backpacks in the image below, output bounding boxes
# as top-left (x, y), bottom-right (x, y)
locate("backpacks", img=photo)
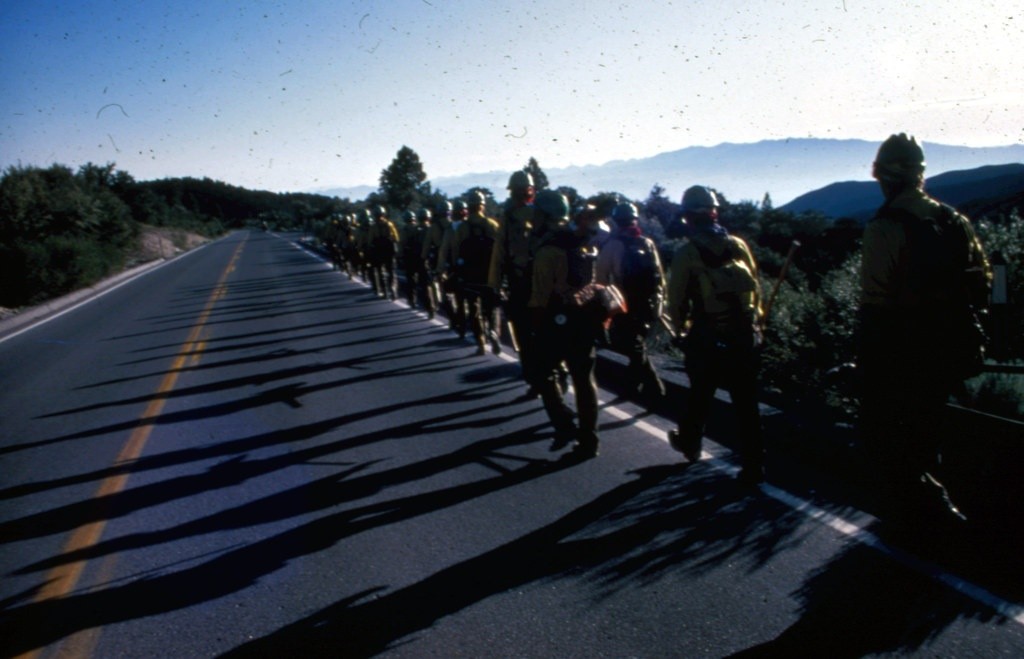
top-left (690, 237), bottom-right (758, 322)
top-left (877, 206), bottom-right (980, 337)
top-left (612, 235), bottom-right (663, 297)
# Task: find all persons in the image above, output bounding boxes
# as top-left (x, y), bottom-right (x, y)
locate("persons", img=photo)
top-left (852, 133), bottom-right (994, 566)
top-left (326, 171), bottom-right (669, 462)
top-left (669, 186), bottom-right (771, 487)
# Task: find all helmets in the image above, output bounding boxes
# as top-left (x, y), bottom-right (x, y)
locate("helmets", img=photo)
top-left (679, 185), bottom-right (721, 208)
top-left (320, 191), bottom-right (485, 223)
top-left (507, 172), bottom-right (535, 188)
top-left (611, 200), bottom-right (640, 220)
top-left (874, 133), bottom-right (923, 174)
top-left (535, 189), bottom-right (571, 218)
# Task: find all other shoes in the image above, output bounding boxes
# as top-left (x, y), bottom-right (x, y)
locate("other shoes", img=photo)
top-left (572, 443), bottom-right (596, 458)
top-left (328, 265), bottom-right (502, 356)
top-left (526, 382), bottom-right (539, 399)
top-left (559, 367), bottom-right (568, 394)
top-left (731, 453), bottom-right (768, 478)
top-left (635, 381), bottom-right (668, 403)
top-left (932, 507), bottom-right (968, 539)
top-left (554, 427), bottom-right (575, 441)
top-left (668, 428), bottom-right (700, 463)
